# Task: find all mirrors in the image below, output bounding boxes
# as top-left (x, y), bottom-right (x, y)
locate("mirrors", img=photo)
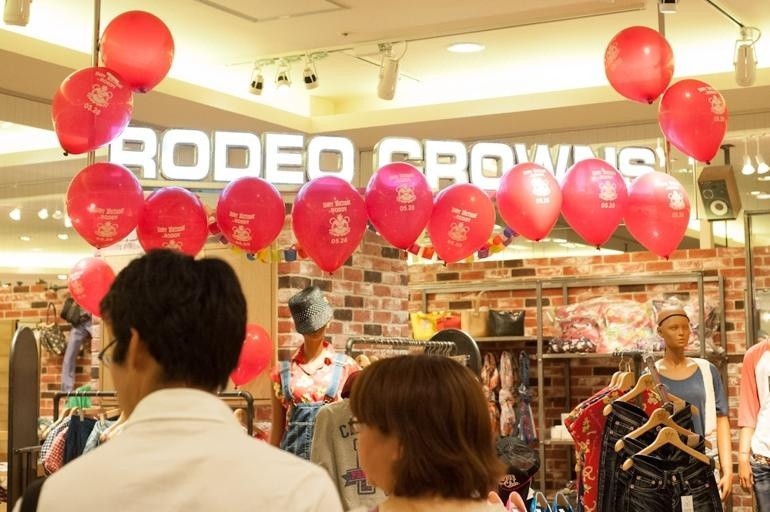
top-left (7, 325), bottom-right (42, 512)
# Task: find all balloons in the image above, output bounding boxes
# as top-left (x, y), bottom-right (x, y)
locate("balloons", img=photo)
top-left (561, 159), bottom-right (629, 247)
top-left (66, 162), bottom-right (146, 249)
top-left (364, 162), bottom-right (433, 250)
top-left (622, 170), bottom-right (691, 260)
top-left (52, 67), bottom-right (134, 154)
top-left (604, 26), bottom-right (674, 105)
top-left (427, 183), bottom-right (496, 263)
top-left (99, 11), bottom-right (174, 92)
top-left (217, 176), bottom-right (286, 251)
top-left (658, 80), bottom-right (727, 163)
top-left (291, 174), bottom-right (369, 273)
top-left (135, 188), bottom-right (208, 259)
top-left (495, 162), bottom-right (562, 242)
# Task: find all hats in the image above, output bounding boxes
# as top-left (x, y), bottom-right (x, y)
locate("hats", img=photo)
top-left (657, 307), bottom-right (690, 327)
top-left (287, 284), bottom-right (335, 335)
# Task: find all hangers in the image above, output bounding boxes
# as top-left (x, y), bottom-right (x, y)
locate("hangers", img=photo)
top-left (40, 390), bottom-right (264, 468)
top-left (570, 350), bottom-right (721, 470)
top-left (352, 338), bottom-right (471, 372)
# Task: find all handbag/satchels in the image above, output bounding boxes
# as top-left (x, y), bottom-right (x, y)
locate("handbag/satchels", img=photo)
top-left (409, 308), bottom-right (525, 340)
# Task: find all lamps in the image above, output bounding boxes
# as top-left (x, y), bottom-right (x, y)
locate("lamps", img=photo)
top-left (733, 24), bottom-right (761, 88)
top-left (742, 135), bottom-right (770, 177)
top-left (354, 42), bottom-right (399, 100)
top-left (251, 52), bottom-right (319, 96)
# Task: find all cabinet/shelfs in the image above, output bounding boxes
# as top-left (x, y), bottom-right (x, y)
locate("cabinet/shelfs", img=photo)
top-left (417, 273), bottom-right (728, 497)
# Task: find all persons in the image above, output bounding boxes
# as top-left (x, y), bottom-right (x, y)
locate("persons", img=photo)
top-left (644, 305), bottom-right (733, 512)
top-left (738, 335), bottom-right (769, 511)
top-left (348, 352), bottom-right (511, 512)
top-left (31, 247), bottom-right (345, 511)
top-left (261, 285), bottom-right (359, 462)
